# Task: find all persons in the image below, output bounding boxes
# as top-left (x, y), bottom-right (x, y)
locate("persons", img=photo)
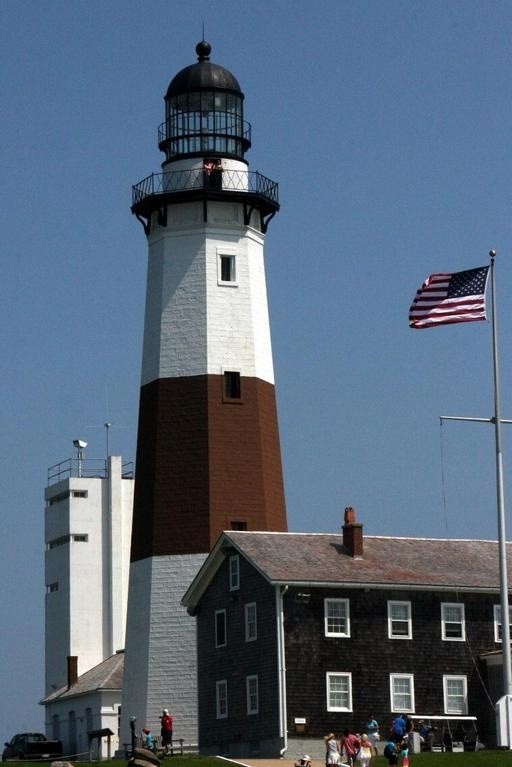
top-left (323, 713), bottom-right (438, 767)
top-left (203, 159), bottom-right (216, 188)
top-left (212, 160), bottom-right (224, 188)
top-left (294, 755), bottom-right (312, 767)
top-left (161, 709), bottom-right (173, 757)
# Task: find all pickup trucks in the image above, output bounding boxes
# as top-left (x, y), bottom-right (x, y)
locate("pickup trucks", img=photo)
top-left (1, 731), bottom-right (65, 764)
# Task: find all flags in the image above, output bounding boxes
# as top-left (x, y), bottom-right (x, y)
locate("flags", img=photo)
top-left (408, 266), bottom-right (491, 329)
